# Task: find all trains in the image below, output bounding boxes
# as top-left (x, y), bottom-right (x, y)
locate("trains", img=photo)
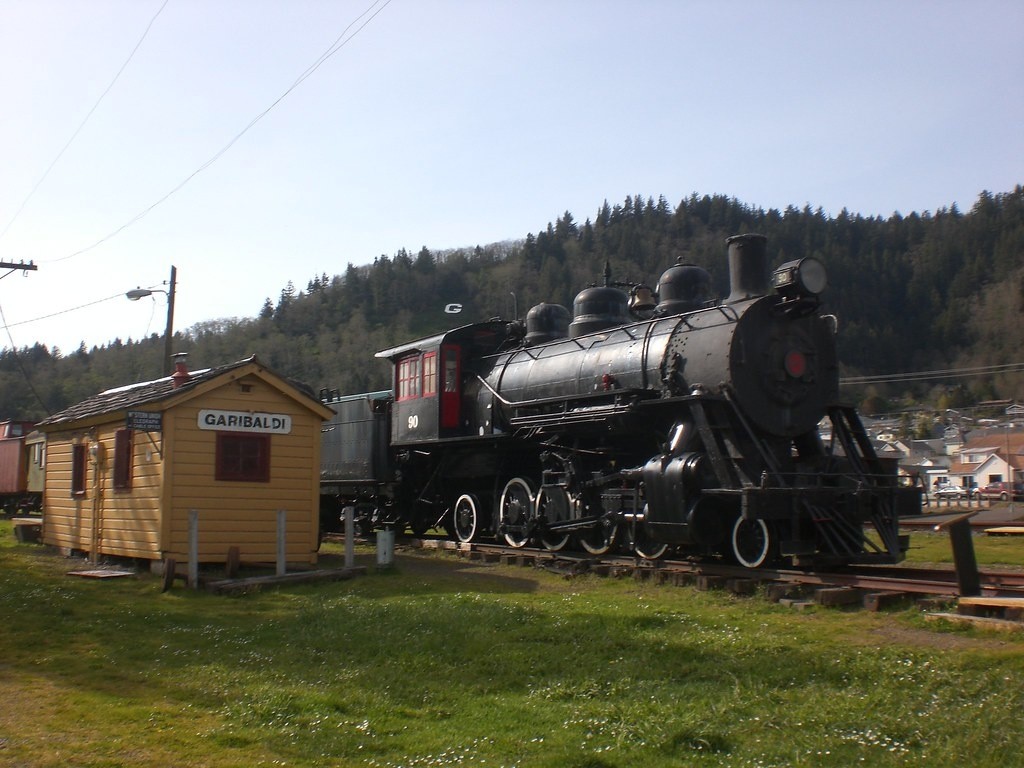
top-left (1, 230), bottom-right (928, 576)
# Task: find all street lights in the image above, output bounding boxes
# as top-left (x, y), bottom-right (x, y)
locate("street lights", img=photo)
top-left (126, 264), bottom-right (177, 377)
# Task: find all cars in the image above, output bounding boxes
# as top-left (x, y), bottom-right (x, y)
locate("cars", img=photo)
top-left (974, 481), bottom-right (1023, 502)
top-left (934, 486), bottom-right (973, 500)
